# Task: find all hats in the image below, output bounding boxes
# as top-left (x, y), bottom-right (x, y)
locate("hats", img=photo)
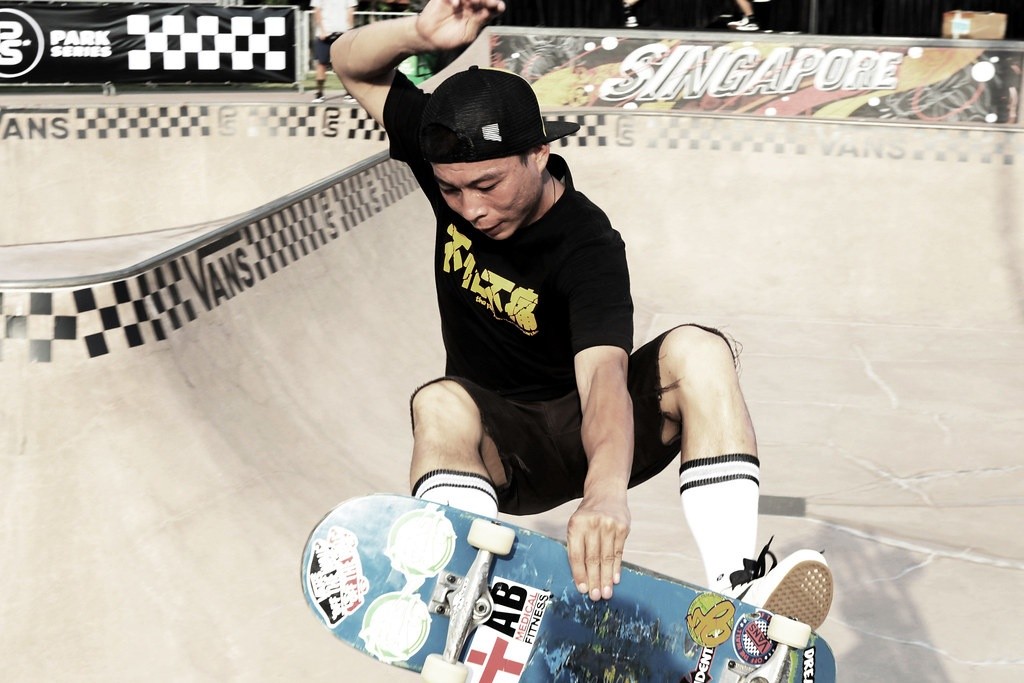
top-left (420, 65), bottom-right (580, 163)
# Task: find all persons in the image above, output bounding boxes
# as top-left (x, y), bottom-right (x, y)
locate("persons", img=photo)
top-left (331, 0), bottom-right (833, 630)
top-left (729, 0), bottom-right (802, 34)
top-left (310, 0), bottom-right (357, 103)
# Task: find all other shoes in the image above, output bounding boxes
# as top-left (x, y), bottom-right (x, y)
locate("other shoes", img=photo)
top-left (312, 94), bottom-right (324, 103)
top-left (727, 16), bottom-right (759, 31)
top-left (624, 9), bottom-right (639, 27)
top-left (342, 95), bottom-right (356, 102)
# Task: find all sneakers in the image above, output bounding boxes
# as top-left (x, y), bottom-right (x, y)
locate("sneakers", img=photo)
top-left (717, 535), bottom-right (833, 632)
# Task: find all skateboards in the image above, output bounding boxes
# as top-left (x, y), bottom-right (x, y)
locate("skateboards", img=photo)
top-left (298, 492), bottom-right (837, 682)
